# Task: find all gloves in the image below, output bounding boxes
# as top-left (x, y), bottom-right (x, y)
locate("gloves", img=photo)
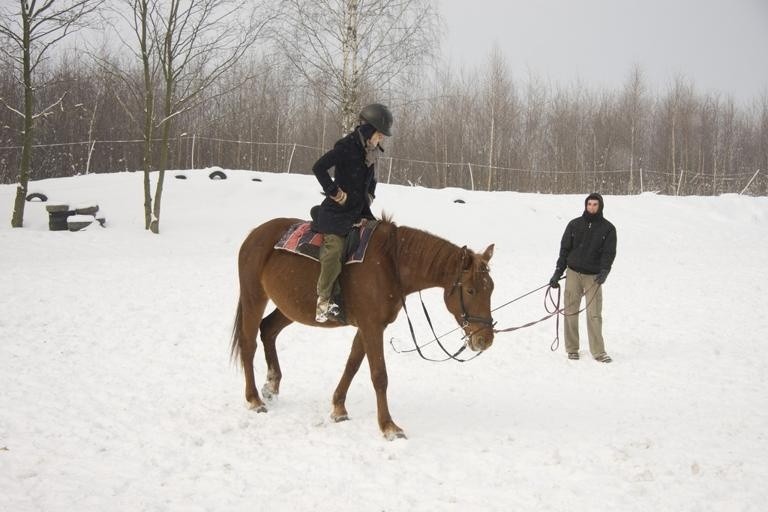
top-left (330, 186), bottom-right (348, 207)
top-left (550, 269), bottom-right (563, 288)
top-left (594, 267), bottom-right (609, 283)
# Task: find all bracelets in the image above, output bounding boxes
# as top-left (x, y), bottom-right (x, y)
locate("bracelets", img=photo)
top-left (334, 191), bottom-right (347, 204)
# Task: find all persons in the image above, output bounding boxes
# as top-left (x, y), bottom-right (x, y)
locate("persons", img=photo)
top-left (547, 192), bottom-right (619, 363)
top-left (310, 102), bottom-right (395, 325)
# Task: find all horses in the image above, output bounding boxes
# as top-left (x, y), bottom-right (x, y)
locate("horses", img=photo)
top-left (228, 211), bottom-right (496, 442)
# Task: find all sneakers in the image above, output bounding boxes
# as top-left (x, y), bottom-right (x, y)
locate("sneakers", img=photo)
top-left (596, 353), bottom-right (611, 362)
top-left (568, 352), bottom-right (579, 359)
top-left (315, 296), bottom-right (341, 323)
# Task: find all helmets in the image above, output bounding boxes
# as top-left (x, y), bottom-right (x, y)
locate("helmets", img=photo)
top-left (360, 104), bottom-right (392, 138)
